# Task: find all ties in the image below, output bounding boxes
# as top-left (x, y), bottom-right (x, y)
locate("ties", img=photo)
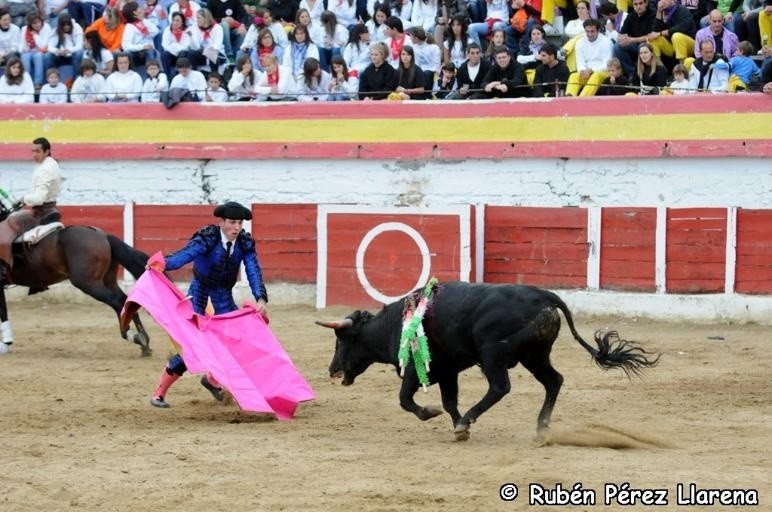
top-left (225, 240), bottom-right (234, 256)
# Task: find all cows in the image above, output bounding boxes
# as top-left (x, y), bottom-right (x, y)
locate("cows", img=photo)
top-left (314, 280), bottom-right (665, 442)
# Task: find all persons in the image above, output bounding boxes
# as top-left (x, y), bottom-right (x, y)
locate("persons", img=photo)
top-left (0, 137), bottom-right (62, 271)
top-left (143, 200), bottom-right (268, 408)
top-left (0, 1), bottom-right (772, 104)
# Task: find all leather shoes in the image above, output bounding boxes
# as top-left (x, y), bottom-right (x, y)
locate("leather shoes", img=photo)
top-left (150, 397), bottom-right (171, 410)
top-left (199, 374), bottom-right (225, 401)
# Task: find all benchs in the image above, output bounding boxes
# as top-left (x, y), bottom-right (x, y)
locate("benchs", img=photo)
top-left (0, 63), bottom-right (151, 84)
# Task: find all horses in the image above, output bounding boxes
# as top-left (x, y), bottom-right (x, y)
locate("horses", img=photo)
top-left (0, 204), bottom-right (153, 358)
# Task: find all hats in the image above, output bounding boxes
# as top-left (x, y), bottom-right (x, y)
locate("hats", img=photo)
top-left (211, 200), bottom-right (254, 221)
